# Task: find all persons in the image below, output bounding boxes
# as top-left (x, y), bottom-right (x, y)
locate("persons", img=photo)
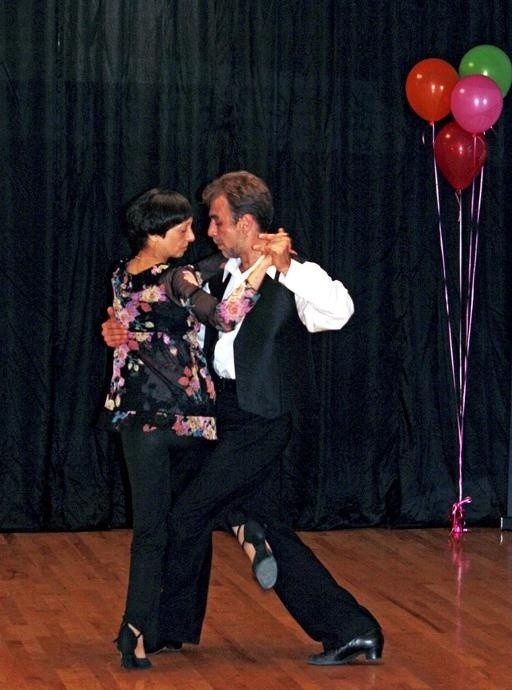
top-left (99, 170), bottom-right (386, 666)
top-left (111, 188), bottom-right (299, 670)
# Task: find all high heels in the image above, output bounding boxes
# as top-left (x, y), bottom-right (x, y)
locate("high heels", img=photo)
top-left (113, 623), bottom-right (152, 669)
top-left (237, 515), bottom-right (278, 590)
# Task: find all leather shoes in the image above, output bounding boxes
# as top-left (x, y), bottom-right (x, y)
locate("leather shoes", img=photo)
top-left (307, 627), bottom-right (384, 666)
top-left (139, 631), bottom-right (183, 656)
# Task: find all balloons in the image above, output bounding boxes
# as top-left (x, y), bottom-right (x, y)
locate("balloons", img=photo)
top-left (405, 44), bottom-right (512, 192)
